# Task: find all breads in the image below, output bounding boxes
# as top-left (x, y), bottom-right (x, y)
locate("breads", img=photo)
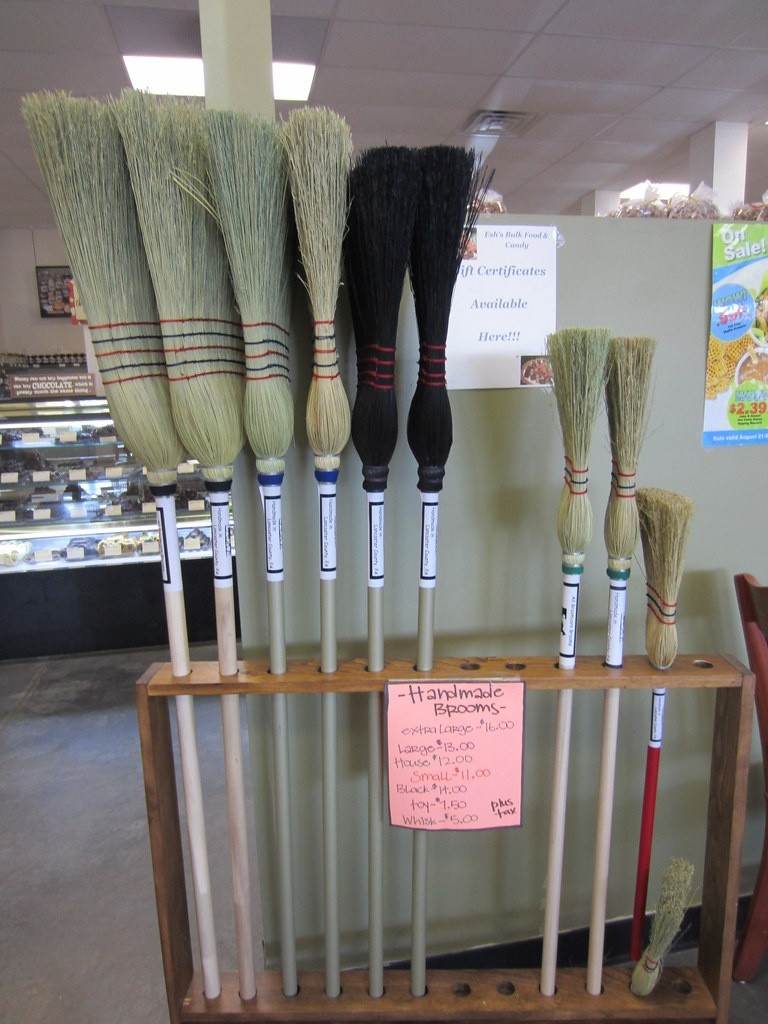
top-left (705, 333), bottom-right (757, 402)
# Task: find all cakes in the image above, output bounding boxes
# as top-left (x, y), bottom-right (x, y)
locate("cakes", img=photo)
top-left (0, 423), bottom-right (212, 565)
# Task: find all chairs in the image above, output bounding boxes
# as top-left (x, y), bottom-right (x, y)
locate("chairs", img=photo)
top-left (731, 573), bottom-right (768, 982)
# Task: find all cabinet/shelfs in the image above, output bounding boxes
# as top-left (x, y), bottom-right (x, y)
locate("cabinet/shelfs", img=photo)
top-left (0, 396), bottom-right (242, 659)
